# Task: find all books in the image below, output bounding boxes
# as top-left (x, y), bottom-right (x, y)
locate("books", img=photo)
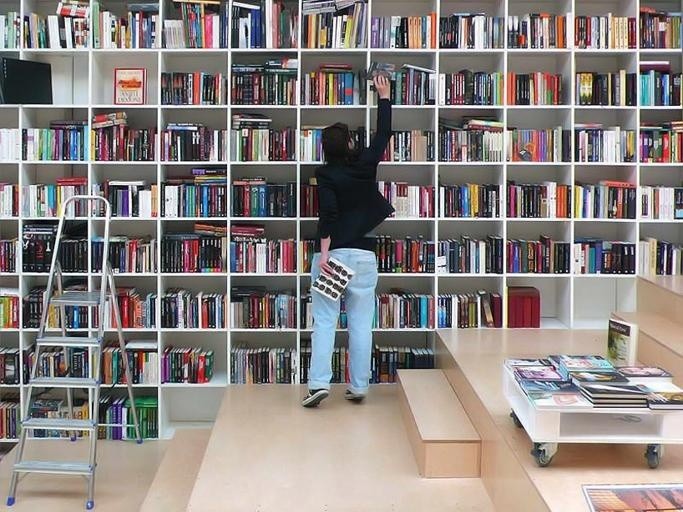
top-left (440, 13), bottom-right (571, 329)
top-left (1, 0), bottom-right (299, 385)
top-left (1, 391), bottom-right (158, 439)
top-left (580, 482), bottom-right (683, 512)
top-left (575, 7), bottom-right (682, 275)
top-left (300, 0), bottom-right (436, 385)
top-left (508, 319), bottom-right (683, 410)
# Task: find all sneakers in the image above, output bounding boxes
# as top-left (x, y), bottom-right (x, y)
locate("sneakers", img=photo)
top-left (302, 389), bottom-right (329, 408)
top-left (345, 389), bottom-right (366, 399)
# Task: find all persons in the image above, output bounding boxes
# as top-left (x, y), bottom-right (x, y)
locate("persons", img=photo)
top-left (304, 75), bottom-right (395, 408)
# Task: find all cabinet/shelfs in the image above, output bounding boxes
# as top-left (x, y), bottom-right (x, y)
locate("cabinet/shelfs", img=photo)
top-left (1, 1), bottom-right (683, 442)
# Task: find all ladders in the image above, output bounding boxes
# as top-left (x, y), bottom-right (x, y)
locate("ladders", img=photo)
top-left (4, 194), bottom-right (142, 511)
